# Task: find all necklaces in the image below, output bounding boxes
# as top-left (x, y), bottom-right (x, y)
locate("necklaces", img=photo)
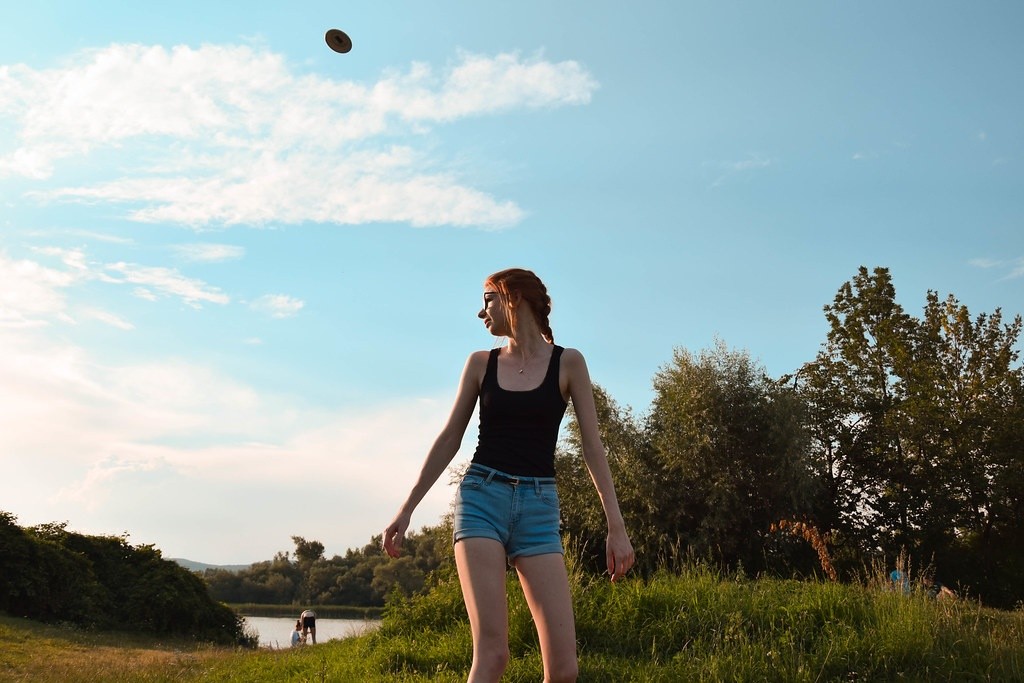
top-left (504, 349), bottom-right (536, 373)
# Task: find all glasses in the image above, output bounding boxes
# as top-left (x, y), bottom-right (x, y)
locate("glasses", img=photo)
top-left (482, 290), bottom-right (508, 310)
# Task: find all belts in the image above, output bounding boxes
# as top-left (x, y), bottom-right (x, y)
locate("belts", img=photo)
top-left (458, 464), bottom-right (558, 486)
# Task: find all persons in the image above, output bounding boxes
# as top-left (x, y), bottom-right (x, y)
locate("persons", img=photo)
top-left (382, 269), bottom-right (634, 683)
top-left (291, 610), bottom-right (317, 645)
